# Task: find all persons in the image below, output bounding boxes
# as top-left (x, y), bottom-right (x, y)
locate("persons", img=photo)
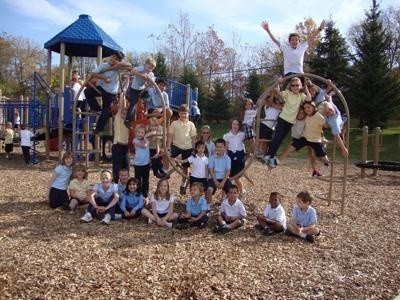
top-left (259, 18), bottom-right (326, 83)
top-left (48, 150), bottom-right (321, 242)
top-left (259, 75), bottom-right (350, 179)
top-left (19, 124), bottom-right (34, 164)
top-left (13, 108), bottom-right (20, 131)
top-left (0, 122), bottom-right (14, 159)
top-left (69, 51), bottom-right (257, 203)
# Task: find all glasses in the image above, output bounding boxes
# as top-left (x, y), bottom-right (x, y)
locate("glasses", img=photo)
top-left (291, 84), bottom-right (300, 87)
top-left (202, 131), bottom-right (209, 134)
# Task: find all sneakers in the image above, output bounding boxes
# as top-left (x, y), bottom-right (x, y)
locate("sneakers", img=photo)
top-left (305, 234), bottom-right (316, 243)
top-left (147, 217), bottom-right (205, 230)
top-left (80, 212), bottom-right (121, 225)
top-left (255, 224), bottom-right (262, 230)
top-left (256, 153), bottom-right (278, 168)
top-left (215, 224), bottom-right (230, 234)
top-left (239, 191), bottom-right (245, 202)
top-left (180, 186), bottom-right (186, 194)
top-left (265, 228), bottom-right (274, 235)
top-left (313, 171), bottom-right (322, 176)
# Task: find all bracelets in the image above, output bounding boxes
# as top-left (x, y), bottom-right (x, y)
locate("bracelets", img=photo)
top-left (318, 27), bottom-right (321, 32)
